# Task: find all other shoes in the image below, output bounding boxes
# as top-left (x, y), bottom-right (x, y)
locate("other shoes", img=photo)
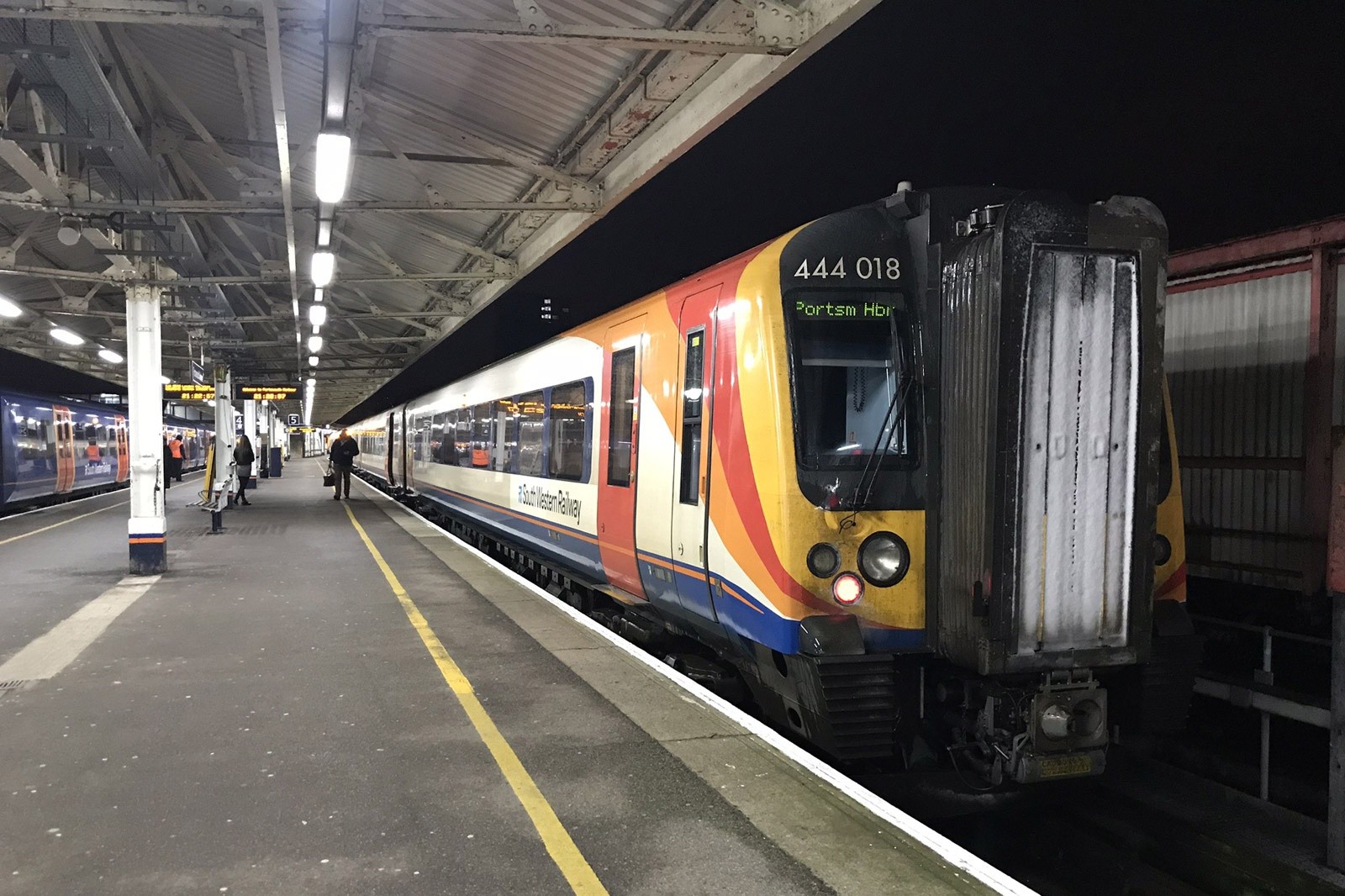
top-left (242, 501), bottom-right (251, 505)
top-left (234, 499), bottom-right (239, 505)
top-left (333, 495), bottom-right (340, 500)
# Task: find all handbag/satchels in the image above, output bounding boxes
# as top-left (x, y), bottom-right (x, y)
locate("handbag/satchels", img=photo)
top-left (324, 466), bottom-right (335, 486)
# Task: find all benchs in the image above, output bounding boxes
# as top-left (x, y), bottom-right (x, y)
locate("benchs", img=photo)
top-left (185, 477), bottom-right (234, 534)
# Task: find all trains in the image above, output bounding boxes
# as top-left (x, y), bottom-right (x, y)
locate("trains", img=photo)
top-left (323, 182), bottom-right (1192, 822)
top-left (0, 381), bottom-right (246, 518)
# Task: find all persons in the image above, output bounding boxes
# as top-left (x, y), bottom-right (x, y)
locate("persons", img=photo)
top-left (328, 429), bottom-right (359, 500)
top-left (169, 435), bottom-right (188, 482)
top-left (189, 437), bottom-right (195, 460)
top-left (75, 422), bottom-right (96, 440)
top-left (163, 435), bottom-right (172, 505)
top-left (37, 420), bottom-right (47, 441)
top-left (233, 435), bottom-right (255, 505)
top-left (21, 418), bottom-right (28, 434)
top-left (431, 435), bottom-right (488, 468)
top-left (84, 437), bottom-right (105, 467)
